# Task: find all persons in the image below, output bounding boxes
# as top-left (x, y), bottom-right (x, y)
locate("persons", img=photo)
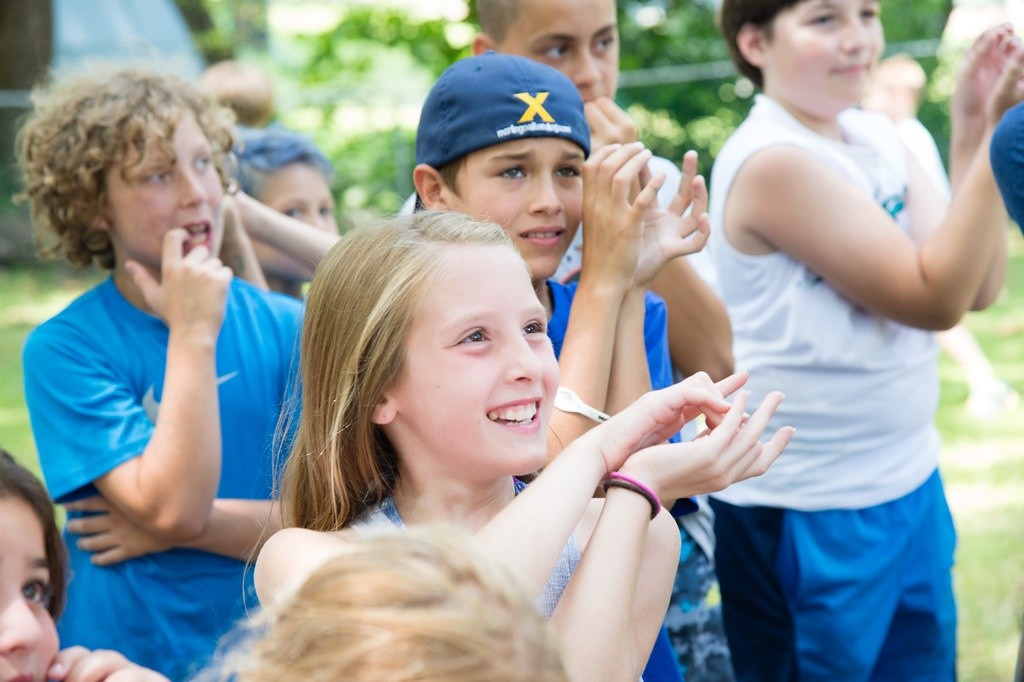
top-left (412, 51), bottom-right (709, 682)
top-left (246, 204), bottom-right (795, 681)
top-left (225, 523), bottom-right (570, 682)
top-left (874, 54), bottom-right (953, 210)
top-left (199, 56), bottom-right (344, 300)
top-left (706, 0), bottom-right (1024, 681)
top-left (11, 67), bottom-right (306, 681)
top-left (396, 0), bottom-right (737, 681)
top-left (0, 446), bottom-right (171, 682)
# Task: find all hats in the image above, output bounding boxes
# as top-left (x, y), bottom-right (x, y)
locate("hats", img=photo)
top-left (412, 50), bottom-right (591, 209)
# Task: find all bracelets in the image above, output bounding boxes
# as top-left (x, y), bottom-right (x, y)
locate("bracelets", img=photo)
top-left (604, 469), bottom-right (661, 520)
